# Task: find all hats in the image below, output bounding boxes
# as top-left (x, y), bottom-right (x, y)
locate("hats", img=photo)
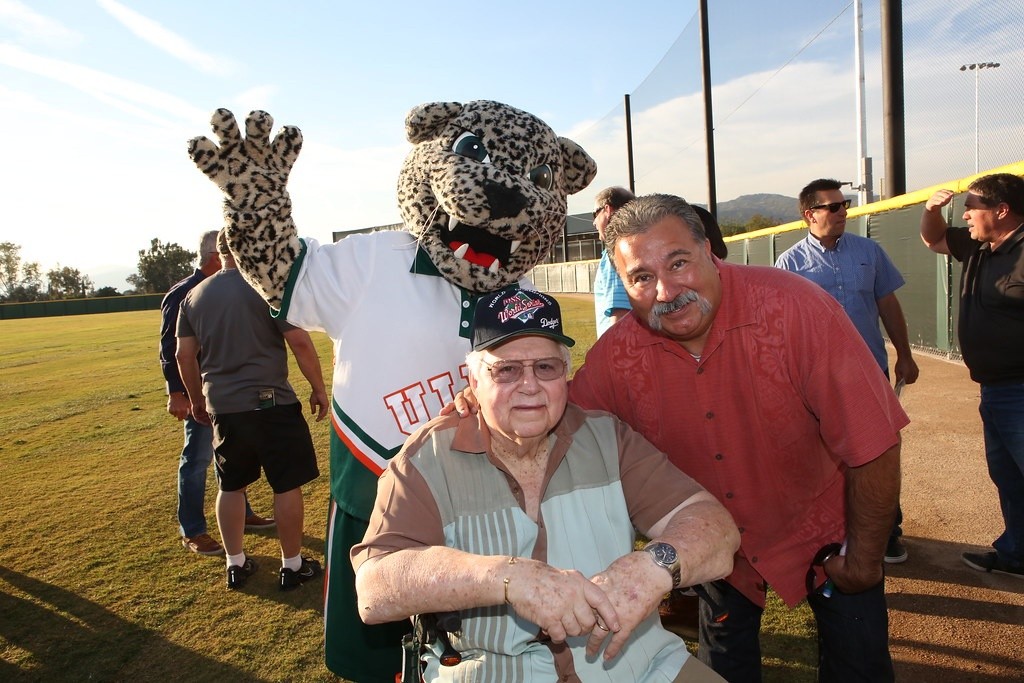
top-left (471, 288), bottom-right (575, 351)
top-left (215, 226), bottom-right (230, 254)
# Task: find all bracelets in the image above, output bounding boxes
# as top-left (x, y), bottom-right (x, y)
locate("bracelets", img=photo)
top-left (503, 555), bottom-right (517, 605)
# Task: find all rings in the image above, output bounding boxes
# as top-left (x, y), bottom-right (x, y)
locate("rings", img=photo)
top-left (596, 620), bottom-right (609, 631)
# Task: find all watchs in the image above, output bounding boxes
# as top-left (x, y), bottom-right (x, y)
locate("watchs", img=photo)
top-left (639, 541), bottom-right (682, 595)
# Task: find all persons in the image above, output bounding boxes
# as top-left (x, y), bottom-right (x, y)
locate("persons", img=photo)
top-left (591, 186), bottom-right (637, 339)
top-left (174, 221), bottom-right (330, 590)
top-left (158, 230), bottom-right (275, 554)
top-left (773, 178), bottom-right (919, 563)
top-left (920, 171), bottom-right (1024, 578)
top-left (437, 194), bottom-right (904, 683)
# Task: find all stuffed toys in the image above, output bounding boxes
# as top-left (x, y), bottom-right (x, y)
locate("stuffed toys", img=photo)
top-left (186, 98), bottom-right (595, 683)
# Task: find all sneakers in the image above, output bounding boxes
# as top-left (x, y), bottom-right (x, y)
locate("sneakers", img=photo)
top-left (278, 556), bottom-right (321, 591)
top-left (245, 512), bottom-right (277, 529)
top-left (226, 557), bottom-right (256, 589)
top-left (181, 532), bottom-right (223, 555)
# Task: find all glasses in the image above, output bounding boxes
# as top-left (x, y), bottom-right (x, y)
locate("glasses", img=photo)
top-left (809, 199), bottom-right (851, 213)
top-left (592, 206), bottom-right (605, 218)
top-left (479, 357), bottom-right (568, 385)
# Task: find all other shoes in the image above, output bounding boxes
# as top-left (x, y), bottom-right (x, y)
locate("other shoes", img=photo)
top-left (961, 549), bottom-right (1024, 579)
top-left (883, 535), bottom-right (907, 564)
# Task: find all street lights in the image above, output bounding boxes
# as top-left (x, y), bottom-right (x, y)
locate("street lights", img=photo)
top-left (960, 62), bottom-right (1000, 174)
